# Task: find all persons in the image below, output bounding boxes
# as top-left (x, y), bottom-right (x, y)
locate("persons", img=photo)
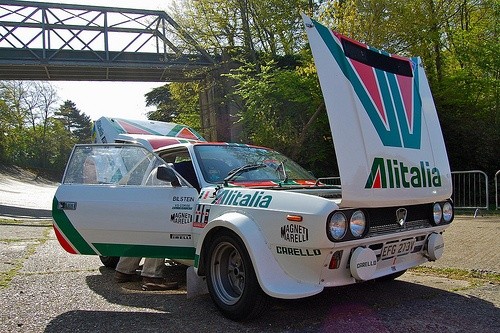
top-left (114, 159), bottom-right (235, 290)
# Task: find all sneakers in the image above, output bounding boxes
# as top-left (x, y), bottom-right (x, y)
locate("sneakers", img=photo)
top-left (140, 277), bottom-right (178, 291)
top-left (114, 271), bottom-right (142, 283)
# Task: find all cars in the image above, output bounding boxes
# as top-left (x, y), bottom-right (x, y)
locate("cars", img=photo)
top-left (52, 11), bottom-right (455, 323)
